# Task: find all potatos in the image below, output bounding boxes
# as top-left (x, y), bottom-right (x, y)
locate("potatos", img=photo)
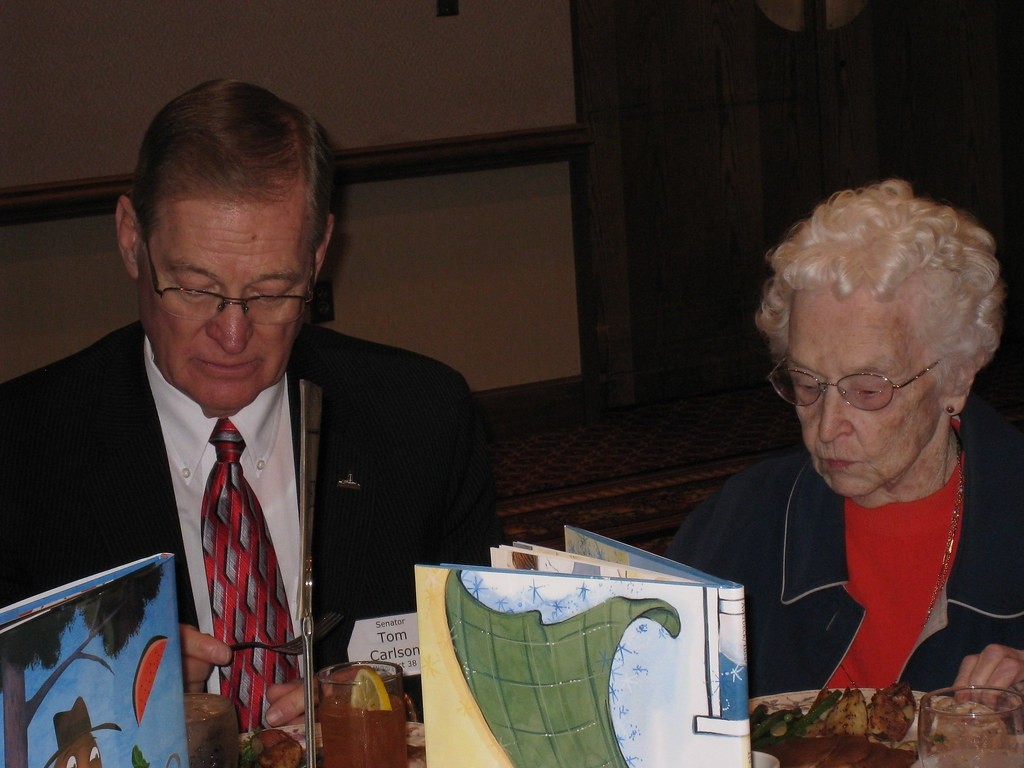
top-left (825, 682), bottom-right (919, 741)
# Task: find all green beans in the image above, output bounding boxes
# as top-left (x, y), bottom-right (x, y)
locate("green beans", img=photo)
top-left (749, 689), bottom-right (843, 750)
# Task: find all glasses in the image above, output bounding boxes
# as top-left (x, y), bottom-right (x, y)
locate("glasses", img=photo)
top-left (128, 193), bottom-right (318, 325)
top-left (768, 354), bottom-right (946, 411)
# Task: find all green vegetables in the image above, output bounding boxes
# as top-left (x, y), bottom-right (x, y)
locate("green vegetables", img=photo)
top-left (238, 726), bottom-right (267, 768)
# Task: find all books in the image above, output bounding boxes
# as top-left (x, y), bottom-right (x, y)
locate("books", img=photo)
top-left (415, 525), bottom-right (752, 768)
top-left (0, 553), bottom-right (190, 768)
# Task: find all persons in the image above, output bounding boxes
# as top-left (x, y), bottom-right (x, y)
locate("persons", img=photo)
top-left (0, 78), bottom-right (510, 730)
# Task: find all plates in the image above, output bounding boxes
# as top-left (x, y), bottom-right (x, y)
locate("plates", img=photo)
top-left (749, 688), bottom-right (1006, 768)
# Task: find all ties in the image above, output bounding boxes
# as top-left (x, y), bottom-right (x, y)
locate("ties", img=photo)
top-left (201, 417), bottom-right (301, 733)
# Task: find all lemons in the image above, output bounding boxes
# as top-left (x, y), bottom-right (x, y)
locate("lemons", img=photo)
top-left (351, 668), bottom-right (392, 710)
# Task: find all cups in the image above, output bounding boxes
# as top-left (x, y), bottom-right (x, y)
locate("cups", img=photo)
top-left (916, 685), bottom-right (1024, 768)
top-left (316, 660), bottom-right (409, 768)
top-left (184, 692), bottom-right (239, 768)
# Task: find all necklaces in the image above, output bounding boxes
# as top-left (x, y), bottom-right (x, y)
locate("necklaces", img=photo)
top-left (840, 449), bottom-right (964, 689)
top-left (652, 181), bottom-right (1024, 711)
